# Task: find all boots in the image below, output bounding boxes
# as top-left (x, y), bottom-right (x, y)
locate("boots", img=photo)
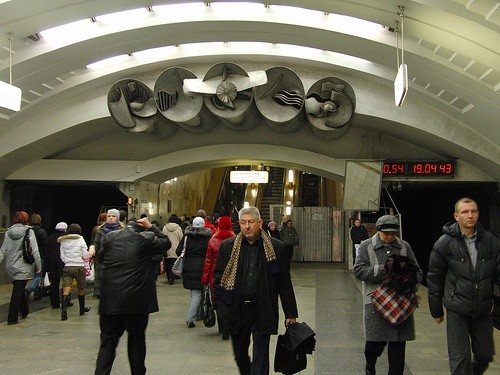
top-left (78, 294), bottom-right (92, 316)
top-left (61, 295), bottom-right (69, 320)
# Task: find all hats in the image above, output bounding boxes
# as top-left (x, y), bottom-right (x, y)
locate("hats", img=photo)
top-left (56, 221), bottom-right (68, 229)
top-left (193, 217), bottom-right (205, 227)
top-left (31, 214), bottom-right (41, 224)
top-left (107, 209), bottom-right (120, 216)
top-left (267, 221), bottom-right (276, 227)
top-left (376, 215), bottom-right (400, 232)
top-left (219, 217), bottom-right (232, 229)
top-left (13, 212), bottom-right (28, 223)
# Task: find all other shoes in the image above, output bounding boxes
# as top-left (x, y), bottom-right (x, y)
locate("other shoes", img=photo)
top-left (25, 289), bottom-right (32, 301)
top-left (222, 328), bottom-right (230, 340)
top-left (7, 321), bottom-right (18, 325)
top-left (34, 289), bottom-right (41, 301)
top-left (186, 321), bottom-right (196, 328)
top-left (52, 305), bottom-right (60, 308)
top-left (21, 312), bottom-right (30, 318)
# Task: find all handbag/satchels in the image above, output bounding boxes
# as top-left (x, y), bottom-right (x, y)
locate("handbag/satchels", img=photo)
top-left (371, 282), bottom-right (421, 325)
top-left (171, 251), bottom-right (185, 278)
top-left (38, 283), bottom-right (51, 297)
top-left (202, 287), bottom-right (216, 327)
top-left (274, 334), bottom-right (307, 375)
top-left (23, 228), bottom-right (34, 264)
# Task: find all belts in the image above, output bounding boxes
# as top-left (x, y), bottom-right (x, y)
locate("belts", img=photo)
top-left (239, 300), bottom-right (257, 306)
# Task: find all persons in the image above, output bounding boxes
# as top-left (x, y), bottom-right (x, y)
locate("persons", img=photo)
top-left (94, 216), bottom-right (172, 375)
top-left (351, 218), bottom-right (369, 266)
top-left (352, 215), bottom-right (424, 375)
top-left (61, 225), bottom-right (92, 320)
top-left (30, 208), bottom-right (126, 308)
top-left (212, 207), bottom-right (297, 375)
top-left (260, 217), bottom-right (299, 272)
top-left (135, 190), bottom-right (240, 341)
top-left (0, 211), bottom-right (42, 325)
top-left (427, 198), bottom-right (500, 375)
